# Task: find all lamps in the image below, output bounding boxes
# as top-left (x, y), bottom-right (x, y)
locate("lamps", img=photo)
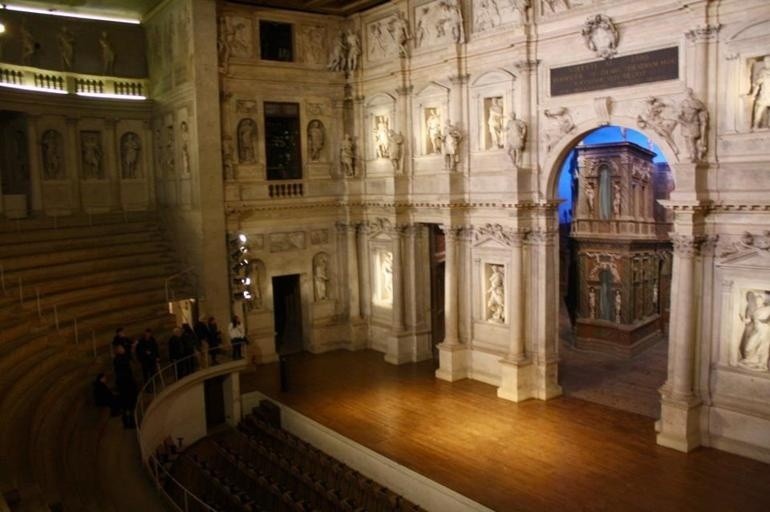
top-left (230, 232), bottom-right (252, 300)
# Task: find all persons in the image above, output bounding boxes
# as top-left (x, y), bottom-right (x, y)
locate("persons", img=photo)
top-left (87, 313), bottom-right (222, 432)
top-left (179, 122), bottom-right (193, 175)
top-left (315, 256), bottom-right (333, 302)
top-left (228, 313), bottom-right (250, 362)
top-left (239, 119), bottom-right (259, 162)
top-left (37, 128), bottom-right (60, 175)
top-left (122, 133), bottom-right (140, 180)
top-left (363, 3), bottom-right (769, 371)
top-left (324, 30), bottom-right (348, 72)
top-left (80, 132), bottom-right (103, 172)
top-left (337, 131), bottom-right (358, 176)
top-left (344, 28), bottom-right (362, 69)
top-left (308, 121), bottom-right (324, 160)
top-left (20, 16), bottom-right (36, 65)
top-left (56, 23), bottom-right (78, 69)
top-left (95, 27), bottom-right (118, 79)
